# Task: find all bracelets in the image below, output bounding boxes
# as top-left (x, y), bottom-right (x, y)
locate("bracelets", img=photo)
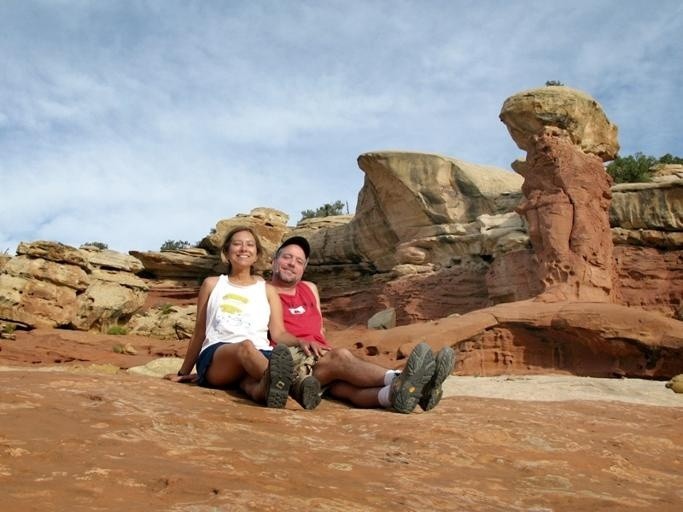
top-left (176, 369), bottom-right (187, 376)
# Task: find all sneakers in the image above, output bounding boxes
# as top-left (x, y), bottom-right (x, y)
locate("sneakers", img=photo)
top-left (418, 346), bottom-right (456, 412)
top-left (389, 343), bottom-right (436, 414)
top-left (263, 342), bottom-right (294, 409)
top-left (291, 374), bottom-right (321, 410)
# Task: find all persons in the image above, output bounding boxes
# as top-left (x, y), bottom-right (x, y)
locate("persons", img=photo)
top-left (162, 226), bottom-right (333, 410)
top-left (171, 236), bottom-right (455, 414)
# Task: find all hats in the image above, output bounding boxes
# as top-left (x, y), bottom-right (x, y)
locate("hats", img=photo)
top-left (277, 236), bottom-right (311, 258)
